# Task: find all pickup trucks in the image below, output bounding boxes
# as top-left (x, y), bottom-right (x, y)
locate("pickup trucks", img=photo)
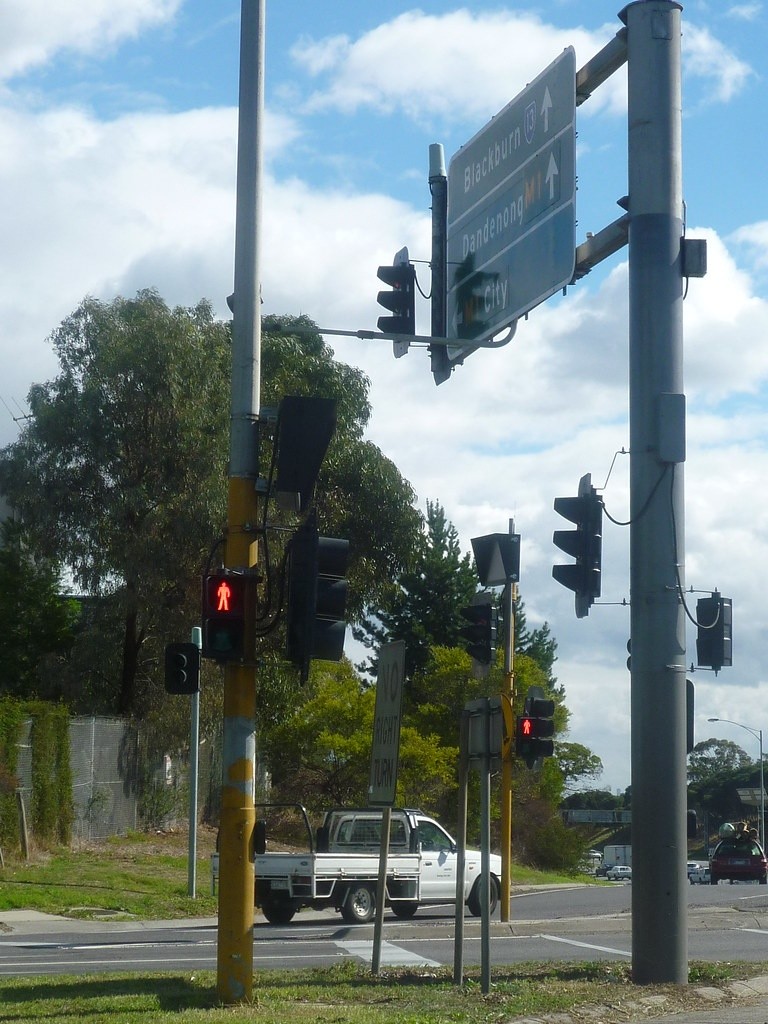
top-left (208, 802), bottom-right (509, 927)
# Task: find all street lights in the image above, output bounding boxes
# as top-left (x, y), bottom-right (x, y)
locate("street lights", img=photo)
top-left (707, 718), bottom-right (765, 852)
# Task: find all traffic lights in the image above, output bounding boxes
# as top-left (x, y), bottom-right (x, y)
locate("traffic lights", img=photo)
top-left (201, 571), bottom-right (244, 659)
top-left (284, 508), bottom-right (352, 679)
top-left (517, 715), bottom-right (538, 738)
top-left (523, 686), bottom-right (556, 770)
top-left (374, 244), bottom-right (416, 358)
top-left (457, 602), bottom-right (497, 665)
top-left (551, 473), bottom-right (596, 619)
top-left (470, 533), bottom-right (522, 587)
top-left (164, 641), bottom-right (197, 695)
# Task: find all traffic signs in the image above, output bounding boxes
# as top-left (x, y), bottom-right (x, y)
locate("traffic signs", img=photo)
top-left (446, 43), bottom-right (577, 360)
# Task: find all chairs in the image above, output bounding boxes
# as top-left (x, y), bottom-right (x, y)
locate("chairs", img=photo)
top-left (389, 826), bottom-right (407, 844)
top-left (350, 824), bottom-right (381, 844)
top-left (719, 845), bottom-right (730, 855)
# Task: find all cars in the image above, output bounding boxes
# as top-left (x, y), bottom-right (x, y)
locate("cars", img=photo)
top-left (687, 862), bottom-right (711, 885)
top-left (595, 863), bottom-right (615, 877)
top-left (708, 838), bottom-right (768, 885)
top-left (605, 865), bottom-right (632, 881)
